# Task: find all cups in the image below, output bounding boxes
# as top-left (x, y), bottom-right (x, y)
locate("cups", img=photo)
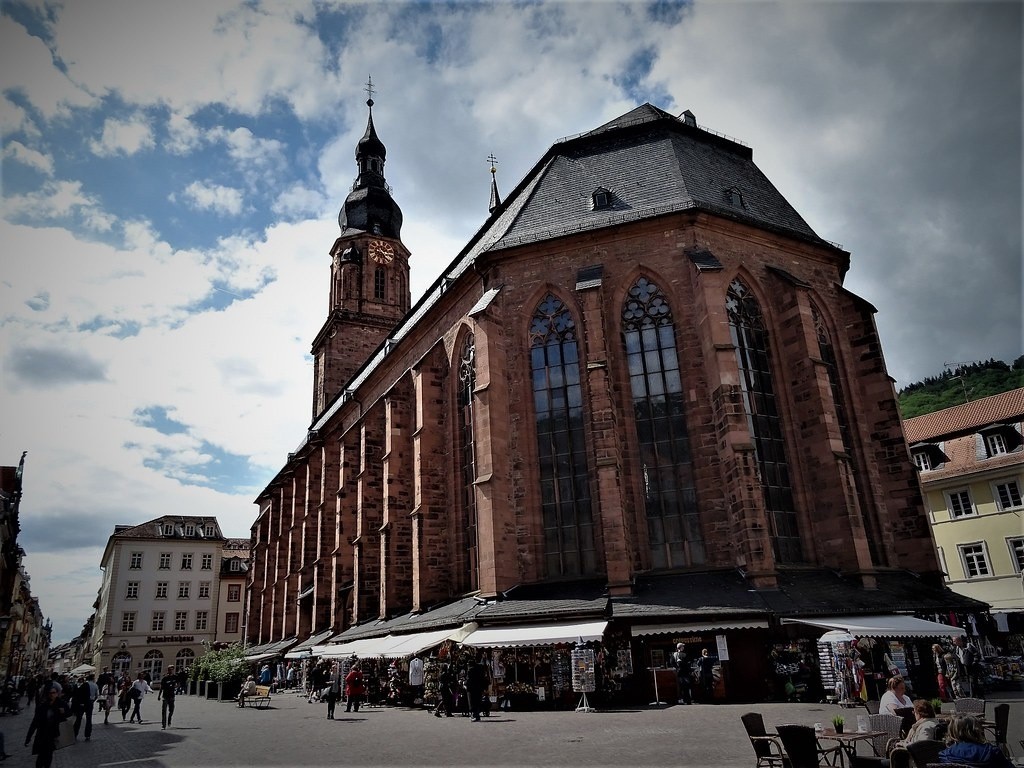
top-left (814, 720), bottom-right (821, 731)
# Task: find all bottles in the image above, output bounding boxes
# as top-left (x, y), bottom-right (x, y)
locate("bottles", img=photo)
top-left (884, 653), bottom-right (902, 678)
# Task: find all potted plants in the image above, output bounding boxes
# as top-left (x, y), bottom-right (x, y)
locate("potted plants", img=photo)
top-left (831, 714), bottom-right (846, 733)
top-left (931, 698), bottom-right (942, 714)
top-left (185, 640), bottom-right (253, 701)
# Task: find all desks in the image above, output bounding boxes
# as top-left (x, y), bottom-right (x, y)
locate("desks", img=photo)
top-left (815, 729), bottom-right (887, 768)
top-left (938, 712), bottom-right (985, 725)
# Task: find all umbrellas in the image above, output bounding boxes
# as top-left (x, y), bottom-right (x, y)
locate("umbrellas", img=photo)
top-left (69, 663), bottom-right (96, 674)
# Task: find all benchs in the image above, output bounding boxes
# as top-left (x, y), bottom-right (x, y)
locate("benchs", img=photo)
top-left (244, 684), bottom-right (271, 708)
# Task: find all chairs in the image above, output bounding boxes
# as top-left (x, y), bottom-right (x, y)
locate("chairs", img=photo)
top-left (774, 724), bottom-right (844, 768)
top-left (849, 698), bottom-right (1014, 768)
top-left (741, 713), bottom-right (787, 768)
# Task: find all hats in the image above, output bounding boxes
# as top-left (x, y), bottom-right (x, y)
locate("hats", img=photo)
top-left (677, 643), bottom-right (685, 649)
top-left (264, 665), bottom-right (268, 668)
top-left (913, 700), bottom-right (934, 713)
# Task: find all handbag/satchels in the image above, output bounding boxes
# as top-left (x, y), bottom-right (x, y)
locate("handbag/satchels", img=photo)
top-left (321, 683), bottom-right (331, 699)
top-left (96, 694), bottom-right (108, 702)
top-left (480, 695), bottom-right (492, 712)
top-left (944, 687), bottom-right (955, 698)
top-left (771, 642), bottom-right (813, 698)
top-left (54, 720), bottom-right (76, 750)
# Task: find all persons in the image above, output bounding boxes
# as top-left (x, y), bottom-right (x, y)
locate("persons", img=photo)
top-left (0, 666), bottom-right (156, 740)
top-left (157, 664), bottom-right (181, 730)
top-left (894, 698), bottom-right (942, 768)
top-left (669, 641), bottom-right (714, 705)
top-left (936, 712), bottom-right (1015, 768)
top-left (258, 665), bottom-right (297, 690)
top-left (878, 677), bottom-right (917, 716)
top-left (24, 686), bottom-right (71, 768)
top-left (307, 663), bottom-right (365, 720)
top-left (434, 659), bottom-right (483, 722)
top-left (176, 666), bottom-right (188, 695)
top-left (931, 636), bottom-right (982, 702)
top-left (0, 732), bottom-right (14, 761)
top-left (233, 674), bottom-right (256, 708)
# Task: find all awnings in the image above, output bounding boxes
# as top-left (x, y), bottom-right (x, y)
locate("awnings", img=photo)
top-left (779, 614), bottom-right (969, 640)
top-left (227, 616), bottom-right (609, 661)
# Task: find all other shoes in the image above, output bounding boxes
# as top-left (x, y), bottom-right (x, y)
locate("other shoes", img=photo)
top-left (239, 706), bottom-right (244, 708)
top-left (320, 700), bottom-right (324, 703)
top-left (139, 720), bottom-right (142, 724)
top-left (327, 714), bottom-right (334, 719)
top-left (353, 709), bottom-right (358, 712)
top-left (0, 755), bottom-right (12, 761)
top-left (446, 714), bottom-right (454, 717)
top-left (308, 700), bottom-right (312, 703)
top-left (234, 697), bottom-right (239, 702)
top-left (344, 710), bottom-right (351, 712)
top-left (435, 713), bottom-right (442, 718)
top-left (131, 721), bottom-right (134, 723)
top-left (471, 718), bottom-right (481, 722)
